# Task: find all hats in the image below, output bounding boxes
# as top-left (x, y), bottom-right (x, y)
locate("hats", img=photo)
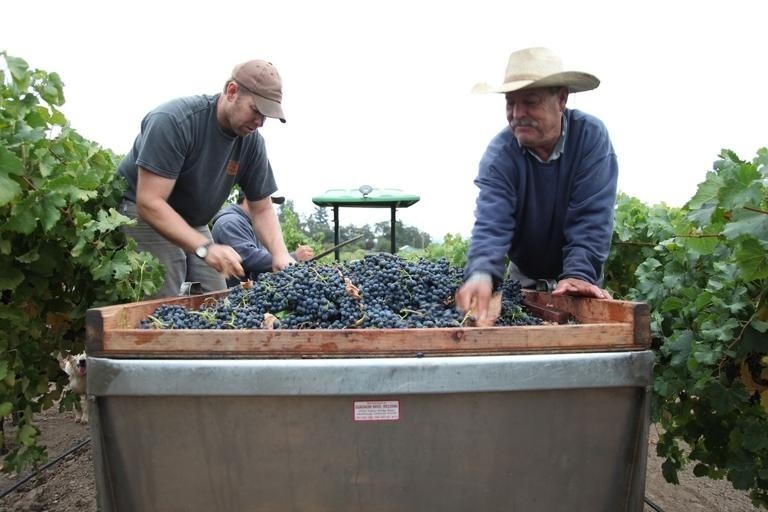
top-left (239, 189), bottom-right (285, 205)
top-left (470, 45), bottom-right (601, 96)
top-left (231, 58), bottom-right (286, 124)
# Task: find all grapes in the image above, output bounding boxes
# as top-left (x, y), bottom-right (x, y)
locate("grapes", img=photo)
top-left (134, 252), bottom-right (543, 329)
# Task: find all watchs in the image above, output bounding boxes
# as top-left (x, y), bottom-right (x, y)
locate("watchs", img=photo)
top-left (193, 242), bottom-right (212, 261)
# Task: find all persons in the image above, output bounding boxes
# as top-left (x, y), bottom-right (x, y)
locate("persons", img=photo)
top-left (453, 85), bottom-right (621, 329)
top-left (114, 59), bottom-right (292, 304)
top-left (209, 187), bottom-right (313, 291)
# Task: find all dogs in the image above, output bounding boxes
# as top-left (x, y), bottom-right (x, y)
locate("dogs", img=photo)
top-left (62, 348), bottom-right (90, 425)
